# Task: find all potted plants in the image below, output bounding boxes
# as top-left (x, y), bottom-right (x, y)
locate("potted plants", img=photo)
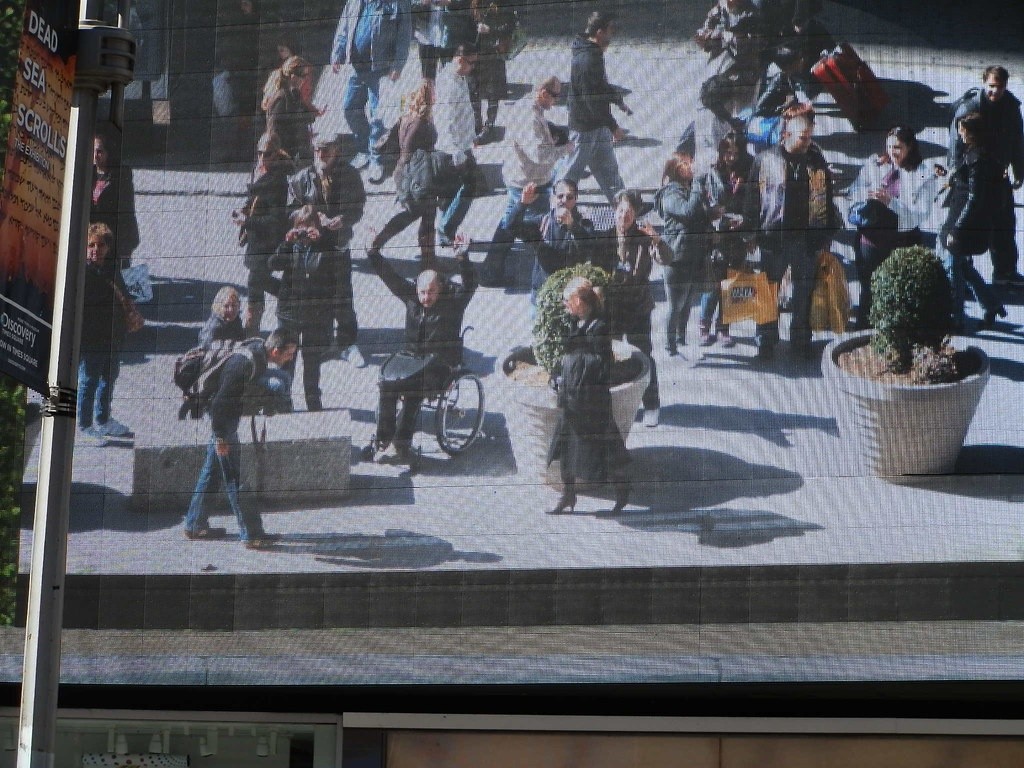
top-left (493, 261), bottom-right (651, 479)
top-left (821, 244), bottom-right (991, 477)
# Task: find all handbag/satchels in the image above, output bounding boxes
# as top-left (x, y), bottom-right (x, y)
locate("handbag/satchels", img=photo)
top-left (120, 264), bottom-right (154, 335)
top-left (615, 199), bottom-right (898, 334)
top-left (452, 11), bottom-right (527, 75)
top-left (701, 3), bottom-right (788, 147)
top-left (374, 117), bottom-right (401, 172)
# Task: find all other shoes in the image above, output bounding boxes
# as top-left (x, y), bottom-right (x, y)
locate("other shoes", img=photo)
top-left (244, 534), bottom-right (283, 550)
top-left (948, 271), bottom-right (1024, 327)
top-left (667, 320), bottom-right (820, 368)
top-left (473, 125), bottom-right (493, 145)
top-left (278, 397), bottom-right (322, 413)
top-left (185, 528), bottom-right (226, 540)
top-left (502, 346), bottom-right (521, 375)
top-left (421, 236), bottom-right (516, 288)
top-left (246, 319), bottom-right (261, 330)
top-left (373, 442), bottom-right (406, 462)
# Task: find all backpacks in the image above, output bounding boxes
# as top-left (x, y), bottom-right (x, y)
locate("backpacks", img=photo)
top-left (172, 338), bottom-right (265, 421)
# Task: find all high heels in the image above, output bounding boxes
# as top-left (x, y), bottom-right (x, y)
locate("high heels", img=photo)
top-left (612, 481), bottom-right (638, 515)
top-left (545, 494), bottom-right (577, 515)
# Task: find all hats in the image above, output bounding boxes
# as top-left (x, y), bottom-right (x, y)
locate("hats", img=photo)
top-left (311, 134), bottom-right (340, 148)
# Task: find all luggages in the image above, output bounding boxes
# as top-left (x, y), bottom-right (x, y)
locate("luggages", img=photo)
top-left (809, 41), bottom-right (885, 134)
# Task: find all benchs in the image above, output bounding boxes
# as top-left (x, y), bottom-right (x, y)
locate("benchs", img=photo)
top-left (133, 409), bottom-right (352, 512)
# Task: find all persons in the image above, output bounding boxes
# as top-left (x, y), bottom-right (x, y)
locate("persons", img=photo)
top-left (78, 0), bottom-right (1023, 552)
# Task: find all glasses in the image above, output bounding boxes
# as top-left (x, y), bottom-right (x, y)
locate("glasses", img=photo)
top-left (544, 89), bottom-right (561, 99)
top-left (258, 151), bottom-right (274, 158)
top-left (557, 194), bottom-right (575, 201)
top-left (298, 73), bottom-right (309, 80)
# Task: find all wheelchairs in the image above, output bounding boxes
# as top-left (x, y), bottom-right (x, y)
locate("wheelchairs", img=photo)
top-left (359, 325), bottom-right (487, 469)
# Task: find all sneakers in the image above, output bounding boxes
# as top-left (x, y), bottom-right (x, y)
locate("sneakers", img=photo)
top-left (78, 416), bottom-right (129, 447)
top-left (341, 345), bottom-right (365, 368)
top-left (644, 409), bottom-right (660, 427)
top-left (349, 151), bottom-right (384, 182)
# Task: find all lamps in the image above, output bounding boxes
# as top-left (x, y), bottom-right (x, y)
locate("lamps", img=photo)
top-left (116, 734), bottom-right (128, 754)
top-left (199, 736), bottom-right (211, 757)
top-left (149, 735), bottom-right (162, 753)
top-left (256, 737), bottom-right (269, 757)
